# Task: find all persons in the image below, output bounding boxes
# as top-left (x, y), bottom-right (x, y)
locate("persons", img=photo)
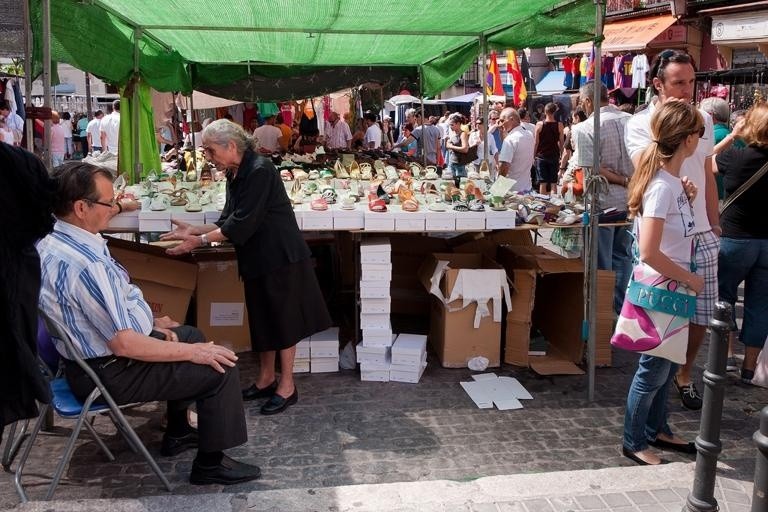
top-left (622, 49), bottom-right (721, 411)
top-left (620, 101), bottom-right (706, 468)
top-left (0, 141), bottom-right (55, 457)
top-left (34, 161), bottom-right (261, 487)
top-left (159, 119), bottom-right (333, 416)
top-left (713, 107), bottom-right (764, 383)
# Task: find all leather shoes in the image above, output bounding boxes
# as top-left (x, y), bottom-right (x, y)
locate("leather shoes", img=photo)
top-left (241, 380), bottom-right (279, 401)
top-left (259, 387), bottom-right (298, 416)
top-left (187, 453), bottom-right (261, 487)
top-left (158, 427), bottom-right (200, 457)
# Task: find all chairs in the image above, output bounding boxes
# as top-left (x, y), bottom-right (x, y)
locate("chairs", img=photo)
top-left (14, 308), bottom-right (173, 505)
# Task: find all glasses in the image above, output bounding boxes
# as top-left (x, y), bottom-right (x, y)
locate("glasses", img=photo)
top-left (474, 115), bottom-right (505, 127)
top-left (656, 50), bottom-right (674, 77)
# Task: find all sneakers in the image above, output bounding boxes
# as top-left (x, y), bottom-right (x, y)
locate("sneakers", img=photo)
top-left (646, 430), bottom-right (698, 455)
top-left (740, 370), bottom-right (756, 385)
top-left (670, 373), bottom-right (705, 414)
top-left (726, 355), bottom-right (738, 372)
top-left (622, 442), bottom-right (671, 466)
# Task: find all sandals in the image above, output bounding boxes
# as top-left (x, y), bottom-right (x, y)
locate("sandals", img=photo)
top-left (109, 142), bottom-right (629, 226)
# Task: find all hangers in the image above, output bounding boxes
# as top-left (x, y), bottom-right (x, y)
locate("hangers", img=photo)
top-left (566, 51), bottom-right (643, 60)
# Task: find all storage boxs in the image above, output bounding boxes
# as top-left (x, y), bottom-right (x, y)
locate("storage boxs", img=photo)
top-left (106, 235), bottom-right (197, 327)
top-left (492, 232), bottom-right (617, 378)
top-left (107, 177), bottom-right (517, 231)
top-left (423, 251), bottom-right (518, 369)
top-left (291, 236), bottom-right (431, 384)
top-left (189, 246), bottom-right (256, 353)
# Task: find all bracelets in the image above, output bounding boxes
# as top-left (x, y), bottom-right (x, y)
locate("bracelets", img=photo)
top-left (200, 231), bottom-right (207, 248)
